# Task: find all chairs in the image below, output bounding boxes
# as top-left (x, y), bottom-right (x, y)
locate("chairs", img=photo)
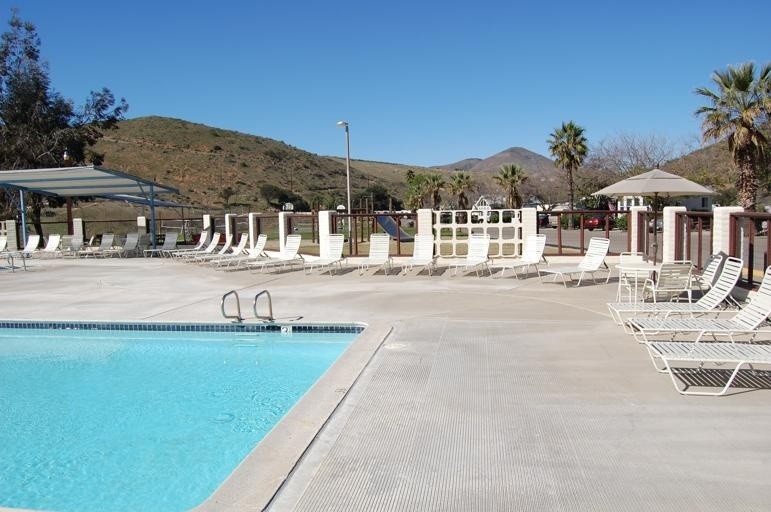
top-left (448, 234), bottom-right (491, 277)
top-left (303, 233), bottom-right (345, 276)
top-left (401, 233), bottom-right (439, 277)
top-left (142, 230), bottom-right (304, 275)
top-left (488, 233), bottom-right (547, 281)
top-left (607, 251), bottom-right (771, 398)
top-left (358, 233), bottom-right (393, 275)
top-left (0, 232), bottom-right (138, 273)
top-left (538, 237), bottom-right (611, 287)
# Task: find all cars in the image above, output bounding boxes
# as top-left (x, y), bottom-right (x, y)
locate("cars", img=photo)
top-left (519, 203), bottom-right (548, 227)
top-left (584, 217), bottom-right (617, 231)
top-left (548, 205), bottom-right (576, 229)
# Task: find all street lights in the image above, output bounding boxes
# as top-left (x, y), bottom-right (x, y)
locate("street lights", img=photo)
top-left (336, 121), bottom-right (353, 256)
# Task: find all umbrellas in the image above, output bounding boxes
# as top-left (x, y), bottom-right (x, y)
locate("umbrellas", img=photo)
top-left (591, 168), bottom-right (722, 284)
